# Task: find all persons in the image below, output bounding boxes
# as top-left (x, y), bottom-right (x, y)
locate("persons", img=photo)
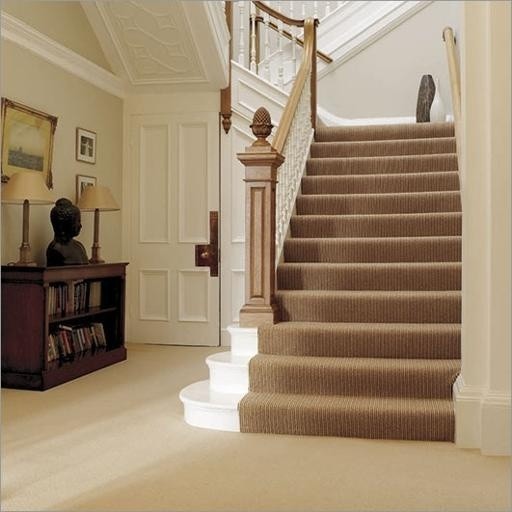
top-left (45, 195), bottom-right (92, 268)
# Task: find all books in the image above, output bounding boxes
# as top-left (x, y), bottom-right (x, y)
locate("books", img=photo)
top-left (47, 282), bottom-right (111, 364)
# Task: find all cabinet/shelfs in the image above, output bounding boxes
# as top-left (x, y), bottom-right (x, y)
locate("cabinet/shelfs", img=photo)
top-left (1, 261), bottom-right (130, 392)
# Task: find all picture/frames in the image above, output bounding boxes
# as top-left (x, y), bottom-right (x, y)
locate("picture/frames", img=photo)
top-left (1, 96), bottom-right (98, 202)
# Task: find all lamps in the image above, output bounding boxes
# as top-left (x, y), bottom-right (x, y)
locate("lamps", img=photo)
top-left (76, 185), bottom-right (120, 264)
top-left (1, 170), bottom-right (55, 268)
